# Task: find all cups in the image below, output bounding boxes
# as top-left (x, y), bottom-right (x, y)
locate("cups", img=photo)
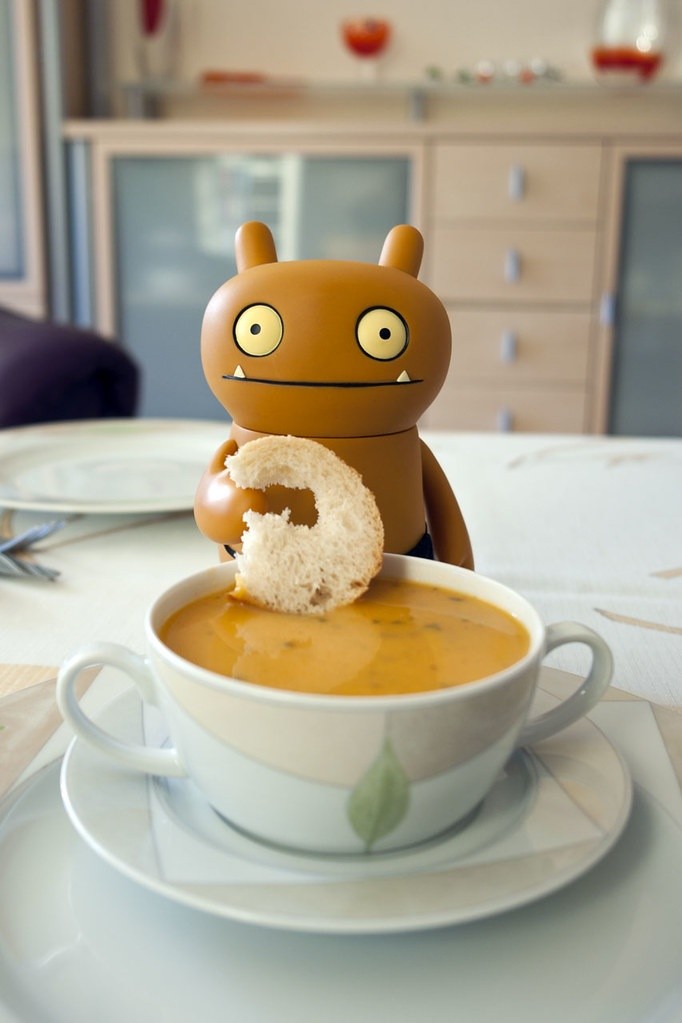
top-left (55, 549), bottom-right (615, 854)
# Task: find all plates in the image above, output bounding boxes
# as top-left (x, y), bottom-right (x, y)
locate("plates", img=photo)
top-left (0, 416), bottom-right (229, 515)
top-left (61, 678), bottom-right (635, 934)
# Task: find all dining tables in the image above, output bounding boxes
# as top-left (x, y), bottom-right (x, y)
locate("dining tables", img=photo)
top-left (0, 434), bottom-right (682, 1023)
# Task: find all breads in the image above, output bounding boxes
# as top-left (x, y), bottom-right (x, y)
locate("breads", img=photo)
top-left (224, 434), bottom-right (384, 614)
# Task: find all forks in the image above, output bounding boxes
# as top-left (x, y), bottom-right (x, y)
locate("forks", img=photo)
top-left (0, 521), bottom-right (64, 583)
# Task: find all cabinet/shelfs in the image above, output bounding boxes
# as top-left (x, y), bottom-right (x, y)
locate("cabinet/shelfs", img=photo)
top-left (63, 84), bottom-right (682, 431)
